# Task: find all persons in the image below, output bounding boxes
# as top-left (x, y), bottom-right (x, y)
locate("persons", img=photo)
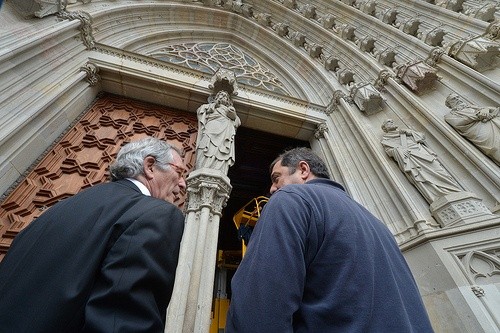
top-left (195, 90), bottom-right (242, 177)
top-left (1, 136), bottom-right (186, 333)
top-left (443, 92), bottom-right (500, 168)
top-left (225, 146), bottom-right (436, 333)
top-left (379, 118), bottom-right (466, 205)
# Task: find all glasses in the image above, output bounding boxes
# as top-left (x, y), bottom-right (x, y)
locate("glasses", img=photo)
top-left (155, 159), bottom-right (186, 177)
